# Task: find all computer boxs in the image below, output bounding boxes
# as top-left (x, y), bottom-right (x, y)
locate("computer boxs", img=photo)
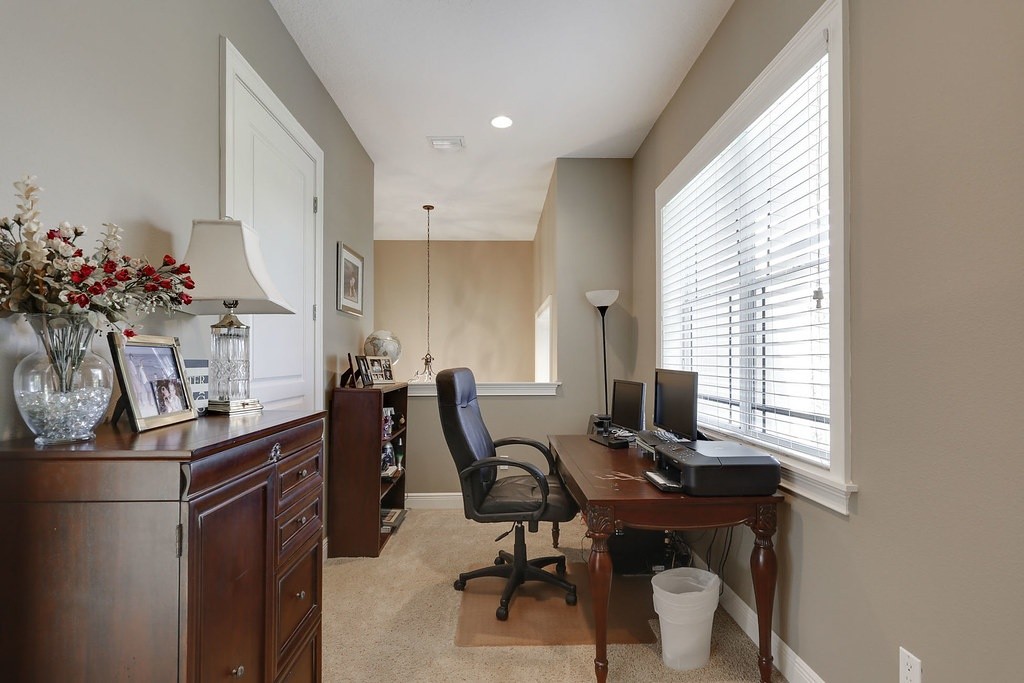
top-left (607, 525), bottom-right (672, 576)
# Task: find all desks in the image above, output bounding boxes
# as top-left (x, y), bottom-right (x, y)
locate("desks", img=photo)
top-left (547, 434), bottom-right (785, 683)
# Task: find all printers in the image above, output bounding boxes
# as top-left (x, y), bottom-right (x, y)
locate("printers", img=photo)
top-left (641, 441), bottom-right (782, 497)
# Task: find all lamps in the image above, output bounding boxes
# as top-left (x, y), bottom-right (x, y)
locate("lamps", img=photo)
top-left (584, 289), bottom-right (620, 415)
top-left (409, 206), bottom-right (447, 386)
top-left (435, 367), bottom-right (578, 620)
top-left (166, 219), bottom-right (294, 415)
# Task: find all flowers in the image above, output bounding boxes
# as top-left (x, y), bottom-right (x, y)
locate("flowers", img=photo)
top-left (0, 177), bottom-right (196, 339)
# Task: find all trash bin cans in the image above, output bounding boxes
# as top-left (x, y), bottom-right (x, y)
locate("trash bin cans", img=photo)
top-left (651, 567), bottom-right (719, 669)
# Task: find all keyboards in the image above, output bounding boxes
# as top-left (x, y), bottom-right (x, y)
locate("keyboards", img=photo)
top-left (589, 436), bottom-right (616, 447)
top-left (638, 431), bottom-right (681, 446)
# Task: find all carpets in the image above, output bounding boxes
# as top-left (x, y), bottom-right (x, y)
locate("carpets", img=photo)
top-left (453, 562), bottom-right (657, 647)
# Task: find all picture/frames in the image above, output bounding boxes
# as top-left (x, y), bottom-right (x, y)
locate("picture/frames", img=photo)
top-left (108, 331), bottom-right (199, 434)
top-left (338, 241), bottom-right (364, 316)
top-left (348, 353), bottom-right (357, 387)
top-left (366, 356), bottom-right (395, 384)
top-left (354, 355), bottom-right (373, 386)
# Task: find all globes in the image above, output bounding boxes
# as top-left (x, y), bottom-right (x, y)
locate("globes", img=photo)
top-left (364, 329), bottom-right (402, 365)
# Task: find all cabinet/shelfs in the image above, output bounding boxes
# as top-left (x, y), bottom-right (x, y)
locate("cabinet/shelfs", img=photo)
top-left (328, 383), bottom-right (407, 557)
top-left (1, 409), bottom-right (329, 683)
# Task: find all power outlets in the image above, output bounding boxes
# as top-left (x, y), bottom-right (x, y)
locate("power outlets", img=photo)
top-left (899, 646), bottom-right (922, 683)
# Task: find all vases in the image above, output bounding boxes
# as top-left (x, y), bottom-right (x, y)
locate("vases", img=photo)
top-left (11, 314), bottom-right (113, 446)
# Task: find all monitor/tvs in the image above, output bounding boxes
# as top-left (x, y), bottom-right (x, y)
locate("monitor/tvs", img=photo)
top-left (652, 369), bottom-right (698, 442)
top-left (610, 379), bottom-right (646, 441)
